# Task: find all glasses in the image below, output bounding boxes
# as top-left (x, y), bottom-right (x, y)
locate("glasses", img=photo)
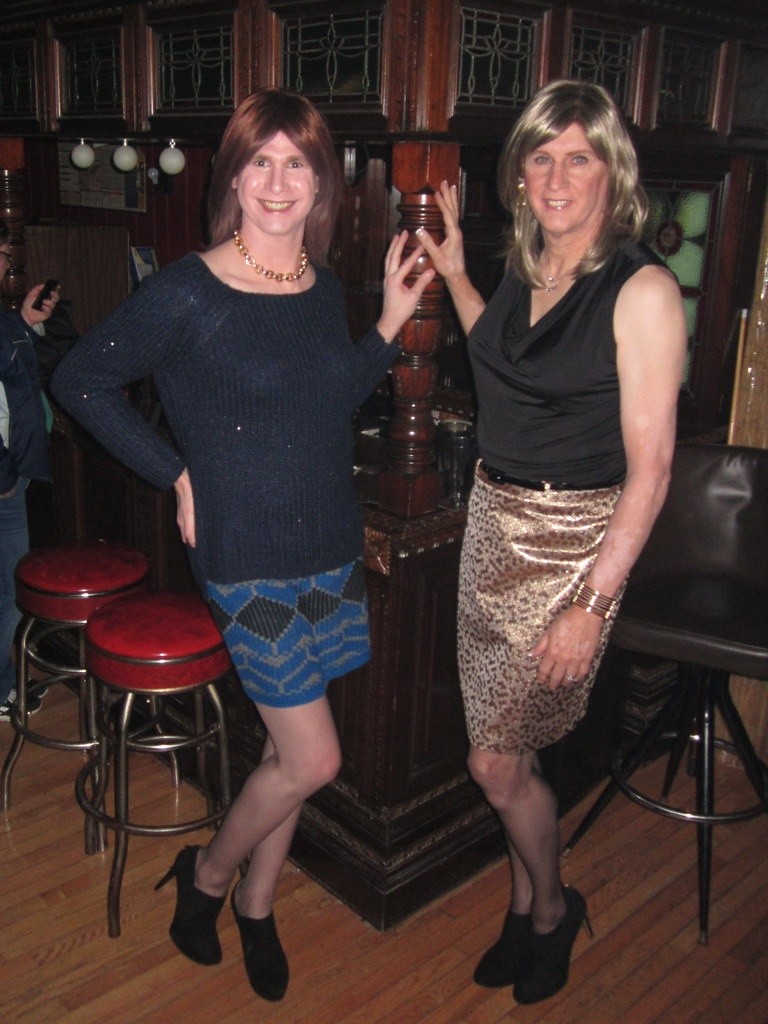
top-left (0, 250), bottom-right (17, 264)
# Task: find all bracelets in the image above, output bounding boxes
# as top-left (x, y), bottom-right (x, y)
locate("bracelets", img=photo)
top-left (572, 582), bottom-right (616, 619)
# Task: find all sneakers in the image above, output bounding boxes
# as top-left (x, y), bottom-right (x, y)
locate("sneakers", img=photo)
top-left (17, 674), bottom-right (48, 698)
top-left (0, 686), bottom-right (43, 722)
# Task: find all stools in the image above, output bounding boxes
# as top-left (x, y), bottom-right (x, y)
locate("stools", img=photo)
top-left (75, 590), bottom-right (249, 940)
top-left (0, 535), bottom-right (180, 855)
top-left (557, 443), bottom-right (768, 947)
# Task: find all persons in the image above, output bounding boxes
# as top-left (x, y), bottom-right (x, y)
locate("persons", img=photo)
top-left (0, 241), bottom-right (63, 719)
top-left (50, 90), bottom-right (432, 1000)
top-left (418, 79), bottom-right (687, 1004)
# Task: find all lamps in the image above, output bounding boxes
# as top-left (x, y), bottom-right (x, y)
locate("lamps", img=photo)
top-left (110, 139), bottom-right (136, 172)
top-left (157, 140), bottom-right (185, 175)
top-left (71, 138), bottom-right (96, 169)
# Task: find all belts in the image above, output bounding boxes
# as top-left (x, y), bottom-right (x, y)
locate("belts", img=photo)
top-left (479, 461), bottom-right (626, 492)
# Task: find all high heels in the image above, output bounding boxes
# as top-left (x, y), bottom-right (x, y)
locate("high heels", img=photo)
top-left (473, 904), bottom-right (533, 987)
top-left (512, 886), bottom-right (594, 1004)
top-left (154, 845), bottom-right (225, 966)
top-left (231, 878), bottom-right (289, 1001)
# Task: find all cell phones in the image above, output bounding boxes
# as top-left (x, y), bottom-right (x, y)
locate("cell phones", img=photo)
top-left (32, 279), bottom-right (59, 311)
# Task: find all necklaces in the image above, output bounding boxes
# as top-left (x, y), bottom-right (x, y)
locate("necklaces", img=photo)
top-left (233, 229), bottom-right (308, 282)
top-left (536, 259), bottom-right (574, 291)
